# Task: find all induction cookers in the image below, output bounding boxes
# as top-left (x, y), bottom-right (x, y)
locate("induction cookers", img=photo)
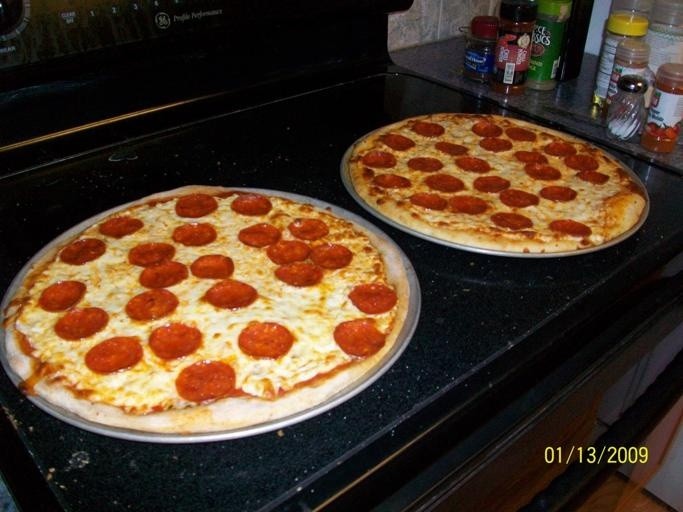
top-left (0, 64), bottom-right (682, 512)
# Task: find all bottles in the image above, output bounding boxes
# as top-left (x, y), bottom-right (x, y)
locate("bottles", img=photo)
top-left (526, 0), bottom-right (573, 93)
top-left (465, 16), bottom-right (499, 80)
top-left (589, 13), bottom-right (649, 117)
top-left (600, 41), bottom-right (650, 128)
top-left (606, 76), bottom-right (646, 142)
top-left (641, 63), bottom-right (682, 154)
top-left (490, 0), bottom-right (537, 96)
top-left (556, 0), bottom-right (596, 83)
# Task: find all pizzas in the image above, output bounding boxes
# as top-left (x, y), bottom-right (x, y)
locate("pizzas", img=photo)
top-left (4, 183), bottom-right (411, 436)
top-left (349, 112), bottom-right (648, 254)
